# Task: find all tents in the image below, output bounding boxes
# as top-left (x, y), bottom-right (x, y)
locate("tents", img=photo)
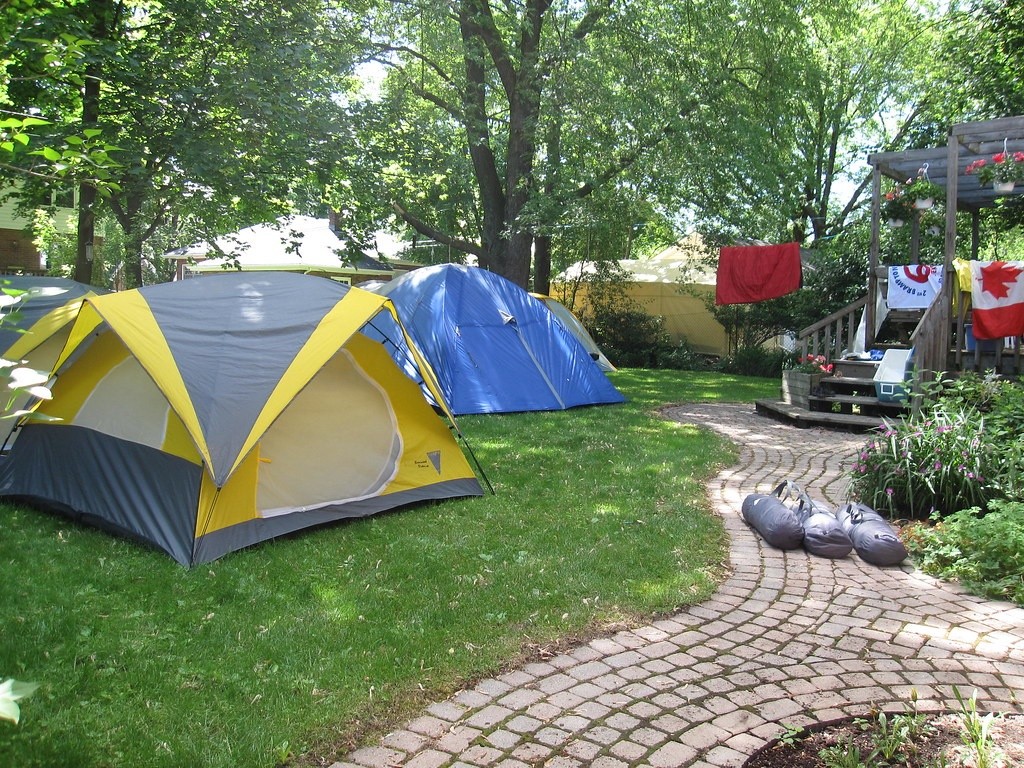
top-left (526, 293), bottom-right (618, 371)
top-left (365, 263), bottom-right (624, 415)
top-left (0, 271), bottom-right (495, 574)
top-left (0, 274), bottom-right (120, 444)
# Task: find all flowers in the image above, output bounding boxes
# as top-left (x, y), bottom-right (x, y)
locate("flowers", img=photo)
top-left (965, 151), bottom-right (1024, 191)
top-left (792, 353), bottom-right (833, 374)
top-left (883, 167), bottom-right (946, 225)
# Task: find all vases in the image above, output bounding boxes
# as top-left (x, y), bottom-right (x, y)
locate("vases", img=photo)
top-left (915, 197), bottom-right (933, 208)
top-left (994, 179), bottom-right (1014, 192)
top-left (782, 370), bottom-right (832, 410)
top-left (888, 218), bottom-right (904, 227)
